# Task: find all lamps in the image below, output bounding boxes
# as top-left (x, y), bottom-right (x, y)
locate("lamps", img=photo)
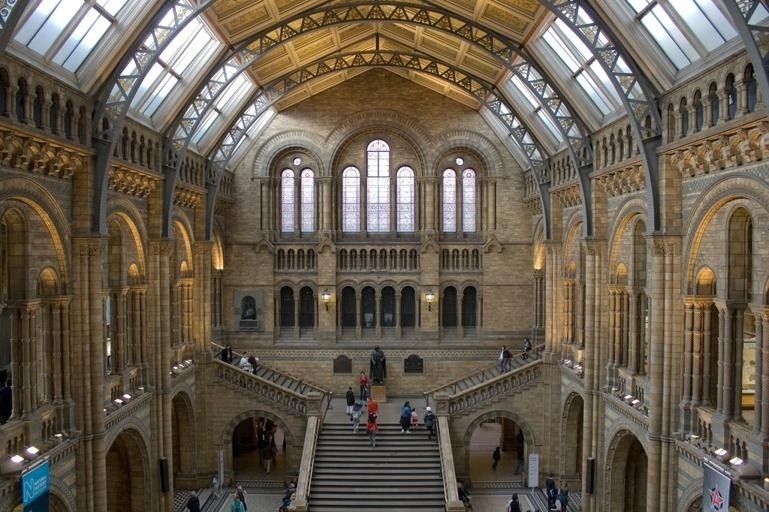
top-left (425, 288), bottom-right (435, 314)
top-left (320, 287), bottom-right (333, 311)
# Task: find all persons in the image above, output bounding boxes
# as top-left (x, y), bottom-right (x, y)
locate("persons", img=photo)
top-left (492, 446), bottom-right (502, 470)
top-left (499, 345), bottom-right (513, 373)
top-left (370, 345), bottom-right (386, 385)
top-left (258, 425), bottom-right (272, 475)
top-left (424, 407), bottom-right (436, 439)
top-left (546, 473), bottom-right (569, 512)
top-left (240, 352), bottom-right (258, 376)
top-left (188, 491), bottom-right (200, 511)
top-left (457, 480), bottom-right (473, 511)
top-left (400, 401), bottom-right (418, 432)
top-left (231, 485), bottom-right (248, 512)
top-left (222, 345), bottom-right (233, 364)
top-left (506, 492), bottom-right (522, 512)
top-left (279, 480), bottom-right (298, 511)
top-left (359, 370), bottom-right (369, 401)
top-left (515, 442), bottom-right (525, 474)
top-left (346, 386), bottom-right (380, 448)
top-left (522, 338), bottom-right (531, 360)
